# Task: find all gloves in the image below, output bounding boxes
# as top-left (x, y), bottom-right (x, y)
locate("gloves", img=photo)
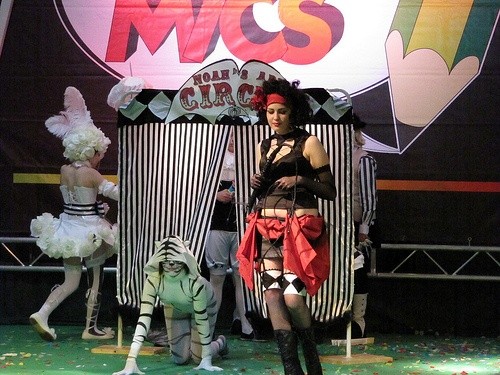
top-left (113, 358), bottom-right (145, 375)
top-left (193, 356), bottom-right (223, 371)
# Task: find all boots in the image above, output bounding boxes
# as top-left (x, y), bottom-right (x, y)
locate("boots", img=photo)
top-left (335, 293), bottom-right (367, 338)
top-left (295, 326), bottom-right (323, 375)
top-left (274, 328), bottom-right (305, 375)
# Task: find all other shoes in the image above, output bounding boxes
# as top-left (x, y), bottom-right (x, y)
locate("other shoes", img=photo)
top-left (217, 335), bottom-right (231, 358)
top-left (30, 313), bottom-right (57, 342)
top-left (82, 327), bottom-right (115, 340)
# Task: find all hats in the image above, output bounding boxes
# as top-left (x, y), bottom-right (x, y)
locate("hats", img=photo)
top-left (352, 112), bottom-right (368, 131)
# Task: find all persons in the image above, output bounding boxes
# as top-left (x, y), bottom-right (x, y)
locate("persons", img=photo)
top-left (30, 86), bottom-right (119, 340)
top-left (205, 132), bottom-right (254, 340)
top-left (350, 113), bottom-right (377, 339)
top-left (112, 235), bottom-right (230, 375)
top-left (235, 79), bottom-right (338, 375)
